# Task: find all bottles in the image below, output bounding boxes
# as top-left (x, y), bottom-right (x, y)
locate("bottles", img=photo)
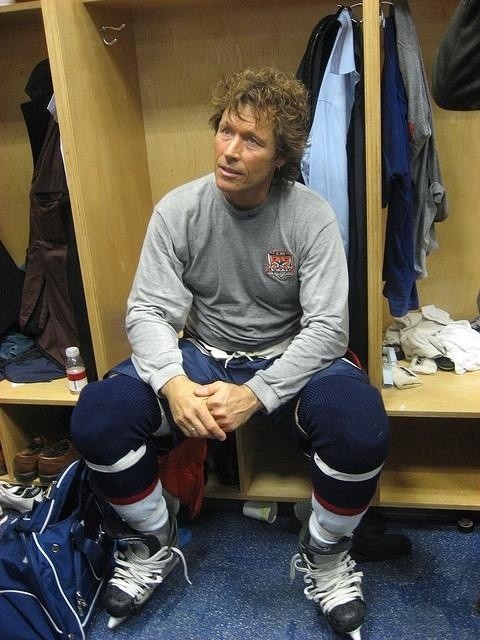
top-left (65, 347), bottom-right (88, 394)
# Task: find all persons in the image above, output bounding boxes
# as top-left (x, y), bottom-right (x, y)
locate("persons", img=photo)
top-left (68, 64), bottom-right (392, 633)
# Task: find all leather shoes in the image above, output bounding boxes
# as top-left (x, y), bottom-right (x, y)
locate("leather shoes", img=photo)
top-left (13, 431), bottom-right (78, 484)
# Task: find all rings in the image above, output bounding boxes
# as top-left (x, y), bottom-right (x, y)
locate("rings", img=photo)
top-left (188, 427), bottom-right (196, 432)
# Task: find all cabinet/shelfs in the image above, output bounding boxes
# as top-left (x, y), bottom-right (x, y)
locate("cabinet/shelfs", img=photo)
top-left (0, 0), bottom-right (480, 512)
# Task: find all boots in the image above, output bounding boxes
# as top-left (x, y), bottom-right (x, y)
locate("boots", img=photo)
top-left (297, 521), bottom-right (369, 633)
top-left (96, 489), bottom-right (183, 619)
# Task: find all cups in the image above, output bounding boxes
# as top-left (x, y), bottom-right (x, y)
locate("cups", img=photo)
top-left (242, 500), bottom-right (278, 524)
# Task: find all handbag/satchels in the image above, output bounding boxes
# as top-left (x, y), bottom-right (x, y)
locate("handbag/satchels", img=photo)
top-left (0, 455), bottom-right (108, 638)
